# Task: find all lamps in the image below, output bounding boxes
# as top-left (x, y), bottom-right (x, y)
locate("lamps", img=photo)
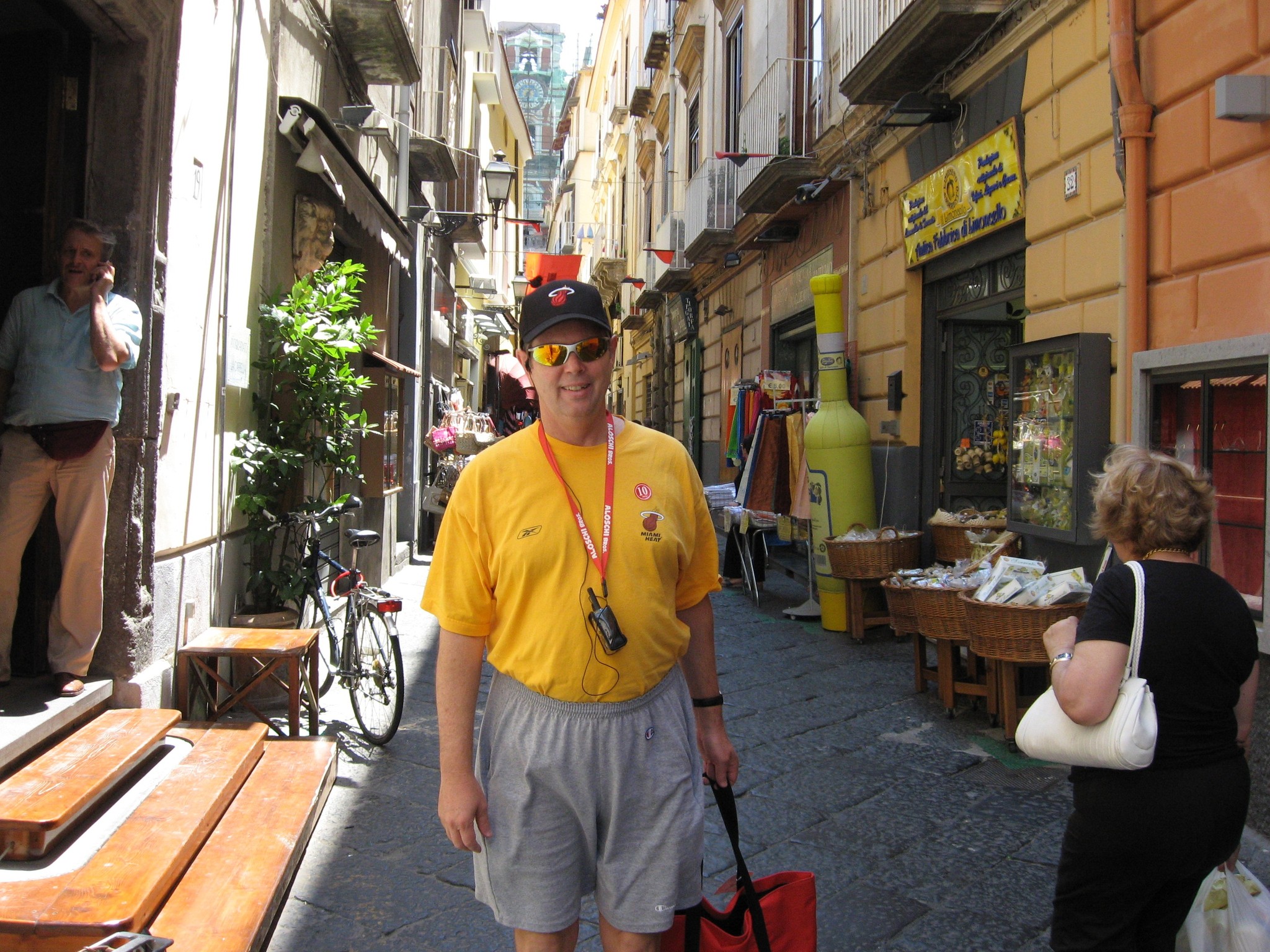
top-left (794, 183), bottom-right (832, 205)
top-left (426, 147), bottom-right (517, 239)
top-left (698, 254), bottom-right (717, 264)
top-left (617, 376), bottom-right (622, 389)
top-left (724, 252), bottom-right (741, 268)
top-left (454, 273), bottom-right (498, 300)
top-left (753, 220), bottom-right (800, 244)
top-left (877, 90), bottom-right (968, 129)
top-left (484, 349), bottom-right (510, 358)
top-left (481, 270), bottom-right (531, 314)
top-left (625, 359), bottom-right (644, 366)
top-left (331, 109), bottom-right (391, 138)
top-left (714, 305), bottom-right (733, 317)
top-left (635, 351), bottom-right (653, 361)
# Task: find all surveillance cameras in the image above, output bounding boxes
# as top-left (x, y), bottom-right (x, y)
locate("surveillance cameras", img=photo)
top-left (793, 182), bottom-right (821, 204)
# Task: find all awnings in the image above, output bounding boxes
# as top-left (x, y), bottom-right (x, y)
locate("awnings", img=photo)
top-left (498, 354), bottom-right (539, 421)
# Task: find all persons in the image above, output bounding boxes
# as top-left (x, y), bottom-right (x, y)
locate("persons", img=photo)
top-left (422, 281), bottom-right (739, 952)
top-left (1044, 444), bottom-right (1258, 952)
top-left (1, 219), bottom-right (144, 696)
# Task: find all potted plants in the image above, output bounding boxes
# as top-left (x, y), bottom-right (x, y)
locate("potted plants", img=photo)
top-left (230, 258), bottom-right (386, 721)
top-left (670, 217), bottom-right (691, 268)
top-left (630, 301), bottom-right (640, 315)
top-left (707, 165), bottom-right (735, 228)
top-left (618, 247), bottom-right (628, 258)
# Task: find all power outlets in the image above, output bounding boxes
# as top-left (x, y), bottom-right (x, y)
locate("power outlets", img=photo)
top-left (880, 419), bottom-right (900, 438)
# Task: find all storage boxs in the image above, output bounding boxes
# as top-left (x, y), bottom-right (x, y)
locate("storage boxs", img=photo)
top-left (972, 554), bottom-right (1095, 605)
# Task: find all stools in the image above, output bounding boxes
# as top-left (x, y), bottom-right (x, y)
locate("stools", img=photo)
top-left (995, 660), bottom-right (1052, 755)
top-left (913, 632), bottom-right (970, 701)
top-left (175, 626), bottom-right (319, 738)
top-left (844, 579), bottom-right (908, 644)
top-left (943, 639), bottom-right (999, 729)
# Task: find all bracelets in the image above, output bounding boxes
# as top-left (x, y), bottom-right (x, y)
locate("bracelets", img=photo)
top-left (1048, 652), bottom-right (1073, 676)
top-left (690, 690), bottom-right (724, 709)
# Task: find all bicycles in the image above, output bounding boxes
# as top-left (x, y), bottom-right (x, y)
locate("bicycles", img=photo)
top-left (258, 495), bottom-right (406, 746)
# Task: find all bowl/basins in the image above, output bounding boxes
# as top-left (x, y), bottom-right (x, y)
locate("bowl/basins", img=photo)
top-left (959, 508), bottom-right (983, 516)
top-left (981, 510), bottom-right (1000, 516)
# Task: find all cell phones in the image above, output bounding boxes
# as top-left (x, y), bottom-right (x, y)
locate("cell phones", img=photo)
top-left (587, 588), bottom-right (627, 652)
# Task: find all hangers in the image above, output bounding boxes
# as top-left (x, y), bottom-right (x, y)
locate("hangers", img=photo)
top-left (762, 399), bottom-right (818, 415)
top-left (732, 378), bottom-right (758, 390)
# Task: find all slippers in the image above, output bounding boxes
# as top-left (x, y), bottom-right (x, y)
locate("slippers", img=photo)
top-left (745, 579), bottom-right (763, 592)
top-left (721, 579), bottom-right (744, 588)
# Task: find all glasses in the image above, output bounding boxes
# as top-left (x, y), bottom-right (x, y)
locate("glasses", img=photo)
top-left (527, 337), bottom-right (611, 367)
top-left (740, 443), bottom-right (743, 446)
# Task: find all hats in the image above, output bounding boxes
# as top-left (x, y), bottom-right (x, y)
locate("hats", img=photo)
top-left (519, 278), bottom-right (612, 346)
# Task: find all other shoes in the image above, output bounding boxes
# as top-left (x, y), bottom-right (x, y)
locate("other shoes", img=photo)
top-left (55, 672), bottom-right (84, 697)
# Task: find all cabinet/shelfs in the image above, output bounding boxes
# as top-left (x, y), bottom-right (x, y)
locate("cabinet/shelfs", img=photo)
top-left (359, 367), bottom-right (406, 499)
top-left (1005, 332), bottom-right (1113, 548)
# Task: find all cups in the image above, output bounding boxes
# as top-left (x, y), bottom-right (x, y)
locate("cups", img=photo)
top-left (985, 452), bottom-right (993, 462)
top-left (984, 463), bottom-right (993, 473)
top-left (955, 447), bottom-right (985, 474)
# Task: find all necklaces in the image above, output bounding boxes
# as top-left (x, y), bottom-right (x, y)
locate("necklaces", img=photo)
top-left (1141, 547), bottom-right (1191, 563)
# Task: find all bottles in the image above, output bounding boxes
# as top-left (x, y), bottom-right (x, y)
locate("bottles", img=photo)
top-left (803, 273), bottom-right (877, 633)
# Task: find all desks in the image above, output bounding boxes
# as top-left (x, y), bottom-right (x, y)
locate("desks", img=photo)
top-left (706, 505), bottom-right (809, 608)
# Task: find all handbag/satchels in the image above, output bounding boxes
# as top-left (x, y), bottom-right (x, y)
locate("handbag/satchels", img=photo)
top-left (424, 412), bottom-right (506, 457)
top-left (25, 421), bottom-right (109, 460)
top-left (422, 465), bottom-right (462, 515)
top-left (1015, 561), bottom-right (1158, 772)
top-left (1175, 860), bottom-right (1270, 952)
top-left (433, 384), bottom-right (464, 418)
top-left (659, 772), bottom-right (817, 951)
